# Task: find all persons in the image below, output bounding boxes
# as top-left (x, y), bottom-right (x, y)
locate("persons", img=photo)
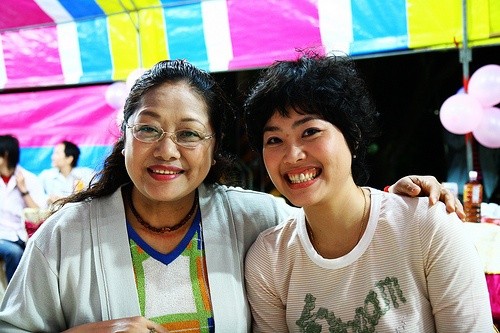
top-left (0, 61), bottom-right (465, 333)
top-left (38, 140), bottom-right (90, 212)
top-left (243, 50), bottom-right (497, 333)
top-left (1, 134), bottom-right (47, 285)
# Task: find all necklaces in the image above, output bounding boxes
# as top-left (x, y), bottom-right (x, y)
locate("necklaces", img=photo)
top-left (128, 183), bottom-right (198, 233)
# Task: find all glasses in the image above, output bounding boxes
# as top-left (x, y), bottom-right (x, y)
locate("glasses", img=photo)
top-left (125, 122), bottom-right (214, 149)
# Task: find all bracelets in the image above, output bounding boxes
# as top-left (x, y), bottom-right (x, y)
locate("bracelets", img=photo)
top-left (21, 190), bottom-right (30, 197)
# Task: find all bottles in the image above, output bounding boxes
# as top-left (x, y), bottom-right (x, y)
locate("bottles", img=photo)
top-left (463, 171), bottom-right (482, 223)
